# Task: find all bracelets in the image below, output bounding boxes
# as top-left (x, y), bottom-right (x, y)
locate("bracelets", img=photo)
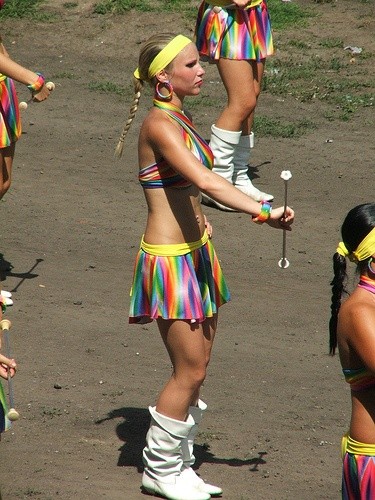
top-left (26, 72), bottom-right (45, 92)
top-left (251, 200), bottom-right (273, 225)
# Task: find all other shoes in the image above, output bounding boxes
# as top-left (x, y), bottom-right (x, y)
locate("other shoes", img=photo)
top-left (1, 290), bottom-right (13, 299)
top-left (2, 297), bottom-right (13, 306)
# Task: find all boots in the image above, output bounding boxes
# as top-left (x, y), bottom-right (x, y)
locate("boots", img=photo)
top-left (141, 405), bottom-right (211, 500)
top-left (182, 398), bottom-right (224, 495)
top-left (232, 132), bottom-right (274, 202)
top-left (201, 124), bottom-right (242, 211)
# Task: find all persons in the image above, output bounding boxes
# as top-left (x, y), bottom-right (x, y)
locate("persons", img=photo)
top-left (0, 294), bottom-right (17, 434)
top-left (196, 0), bottom-right (275, 212)
top-left (117, 31), bottom-right (296, 500)
top-left (328, 202), bottom-right (375, 500)
top-left (0, 35), bottom-right (53, 308)
